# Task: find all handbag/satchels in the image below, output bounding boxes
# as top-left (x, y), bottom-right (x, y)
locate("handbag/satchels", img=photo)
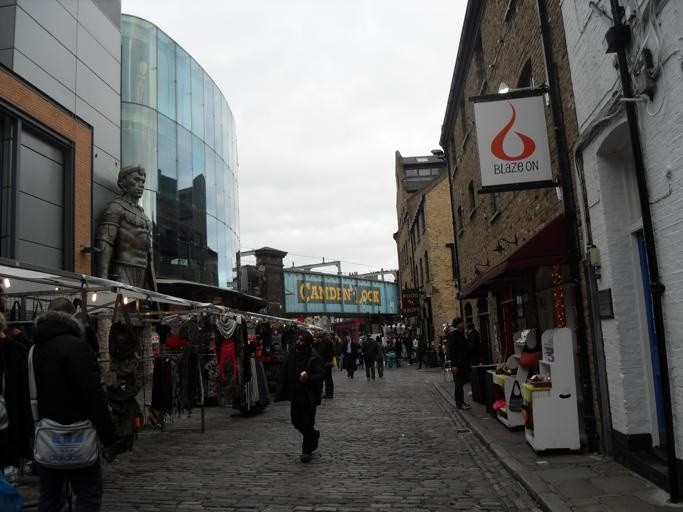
top-left (32, 418), bottom-right (99, 469)
top-left (108, 325), bottom-right (139, 361)
top-left (520, 346), bottom-right (542, 368)
top-left (509, 394), bottom-right (523, 412)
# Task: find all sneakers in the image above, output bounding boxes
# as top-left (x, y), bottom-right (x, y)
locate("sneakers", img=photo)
top-left (301, 431), bottom-right (320, 462)
top-left (456, 402), bottom-right (471, 410)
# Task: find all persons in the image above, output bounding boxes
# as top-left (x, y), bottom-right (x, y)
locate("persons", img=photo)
top-left (333, 333), bottom-right (428, 383)
top-left (95, 164), bottom-right (153, 288)
top-left (447, 317), bottom-right (471, 410)
top-left (317, 332), bottom-right (334, 398)
top-left (16, 296), bottom-right (114, 512)
top-left (283, 330), bottom-right (325, 463)
top-left (464, 322), bottom-right (480, 364)
